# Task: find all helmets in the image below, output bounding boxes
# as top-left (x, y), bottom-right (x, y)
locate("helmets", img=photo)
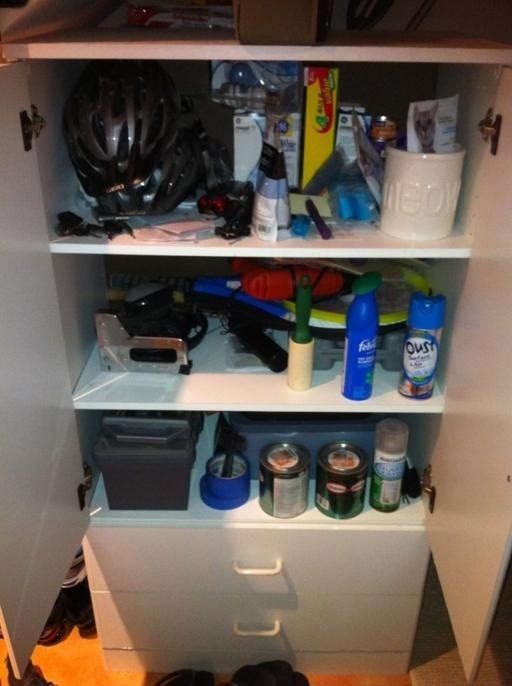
top-left (62, 59), bottom-right (212, 218)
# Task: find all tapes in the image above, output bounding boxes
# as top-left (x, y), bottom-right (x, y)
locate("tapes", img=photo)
top-left (199, 473), bottom-right (250, 510)
top-left (206, 450), bottom-right (250, 499)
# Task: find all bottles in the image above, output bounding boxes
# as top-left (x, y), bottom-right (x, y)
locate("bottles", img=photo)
top-left (259, 441), bottom-right (309, 519)
top-left (399, 291), bottom-right (445, 399)
top-left (369, 418), bottom-right (409, 512)
top-left (315, 442), bottom-right (367, 520)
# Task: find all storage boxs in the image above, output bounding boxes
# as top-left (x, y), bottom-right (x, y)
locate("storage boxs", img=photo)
top-left (229, 412), bottom-right (386, 481)
top-left (92, 431), bottom-right (196, 511)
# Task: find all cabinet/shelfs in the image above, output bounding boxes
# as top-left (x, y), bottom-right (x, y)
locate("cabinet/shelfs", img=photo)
top-left (0, 26), bottom-right (512, 685)
